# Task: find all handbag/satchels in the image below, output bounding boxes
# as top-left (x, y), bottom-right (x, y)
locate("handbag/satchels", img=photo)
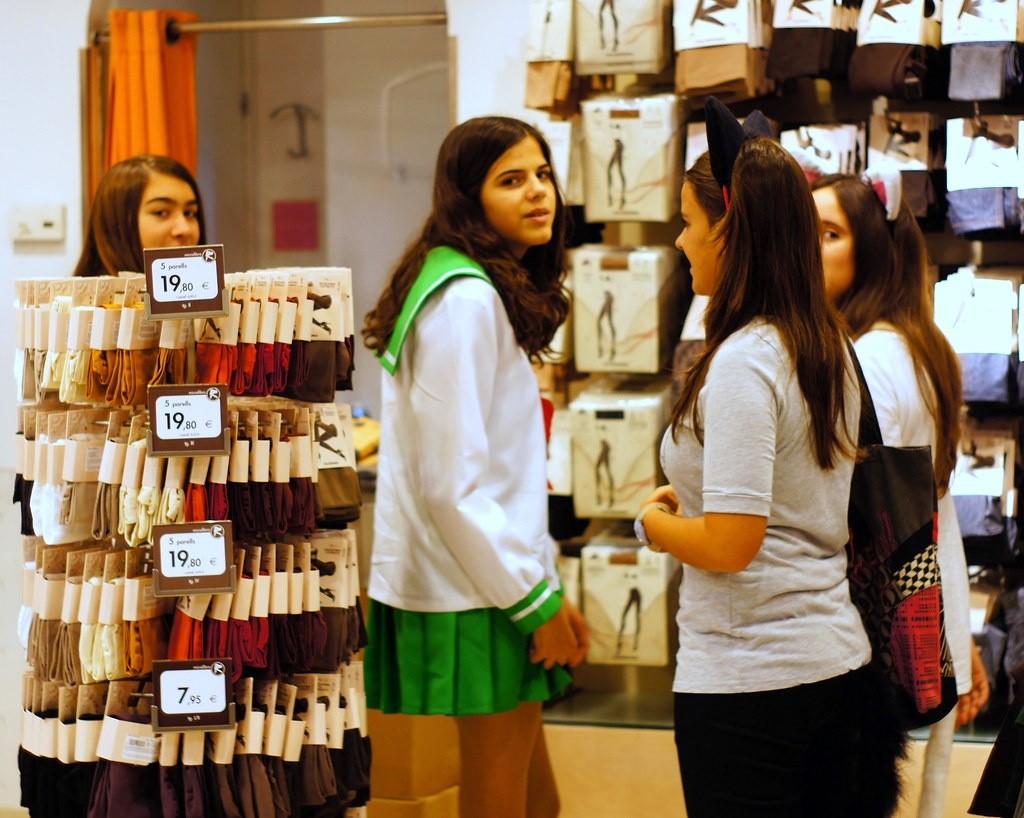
top-left (844, 335), bottom-right (959, 732)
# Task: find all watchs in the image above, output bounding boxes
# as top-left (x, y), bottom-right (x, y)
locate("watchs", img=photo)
top-left (634, 503), bottom-right (668, 546)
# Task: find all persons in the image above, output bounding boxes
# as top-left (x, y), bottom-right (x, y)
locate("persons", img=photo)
top-left (73, 154), bottom-right (208, 276)
top-left (639, 95), bottom-right (989, 818)
top-left (363, 117), bottom-right (590, 818)
